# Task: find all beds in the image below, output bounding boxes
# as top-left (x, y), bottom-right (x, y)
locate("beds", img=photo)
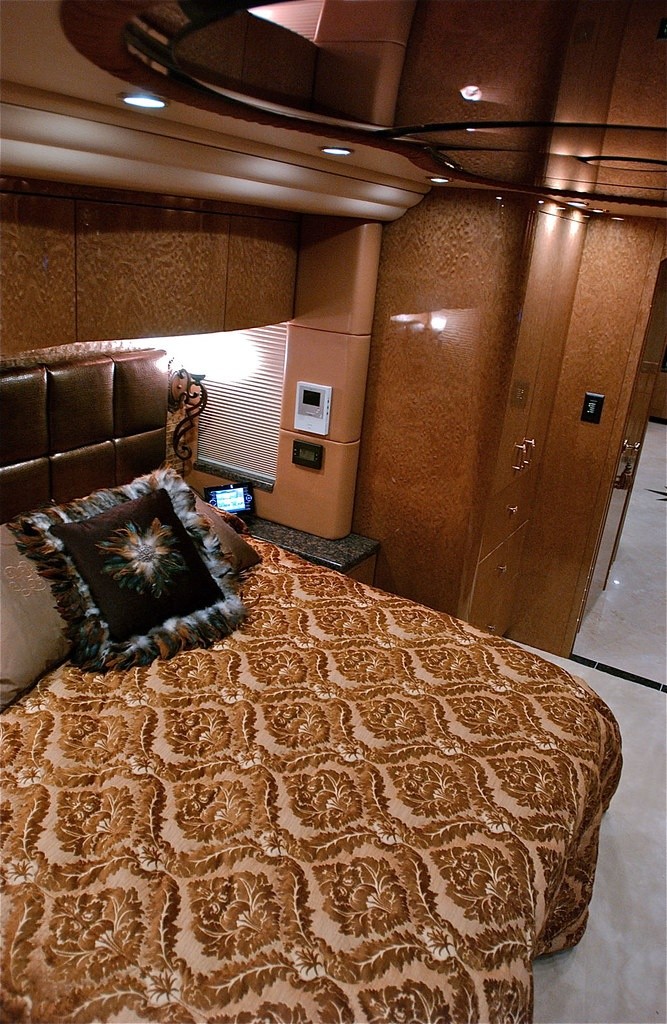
top-left (0, 349), bottom-right (624, 1024)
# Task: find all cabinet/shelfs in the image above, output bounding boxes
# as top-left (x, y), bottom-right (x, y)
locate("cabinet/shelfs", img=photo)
top-left (352, 187), bottom-right (590, 637)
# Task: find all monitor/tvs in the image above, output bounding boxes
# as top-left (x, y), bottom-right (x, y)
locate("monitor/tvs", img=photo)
top-left (204, 483), bottom-right (256, 516)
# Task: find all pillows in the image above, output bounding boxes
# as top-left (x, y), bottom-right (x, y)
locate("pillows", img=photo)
top-left (0, 523), bottom-right (73, 714)
top-left (8, 466), bottom-right (247, 672)
top-left (191, 491), bottom-right (262, 574)
